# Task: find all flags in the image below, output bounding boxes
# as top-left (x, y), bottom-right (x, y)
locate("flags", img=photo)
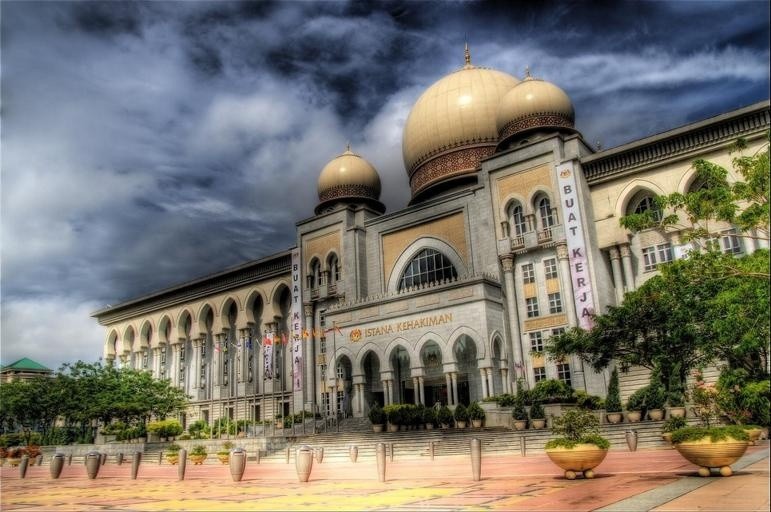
top-left (215, 322), bottom-right (343, 353)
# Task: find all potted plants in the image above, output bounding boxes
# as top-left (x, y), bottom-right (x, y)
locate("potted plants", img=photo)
top-left (217, 451), bottom-right (231, 464)
top-left (529, 392), bottom-right (546, 430)
top-left (604, 364), bottom-right (710, 424)
top-left (166, 444), bottom-right (183, 464)
top-left (741, 423), bottom-right (763, 441)
top-left (187, 411), bottom-right (321, 440)
top-left (189, 445), bottom-right (208, 464)
top-left (545, 435), bottom-right (608, 471)
top-left (660, 415), bottom-right (685, 443)
top-left (368, 402), bottom-right (484, 433)
top-left (672, 425), bottom-right (748, 468)
top-left (512, 378), bottom-right (529, 431)
top-left (116, 416), bottom-right (183, 444)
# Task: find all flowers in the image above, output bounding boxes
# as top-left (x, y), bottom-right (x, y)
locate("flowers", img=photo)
top-left (0, 448), bottom-right (8, 457)
top-left (9, 448), bottom-right (25, 458)
top-left (27, 444), bottom-right (40, 457)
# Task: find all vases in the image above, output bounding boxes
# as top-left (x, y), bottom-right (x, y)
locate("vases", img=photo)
top-left (0, 457), bottom-right (5, 466)
top-left (28, 458), bottom-right (38, 466)
top-left (7, 458), bottom-right (23, 467)
